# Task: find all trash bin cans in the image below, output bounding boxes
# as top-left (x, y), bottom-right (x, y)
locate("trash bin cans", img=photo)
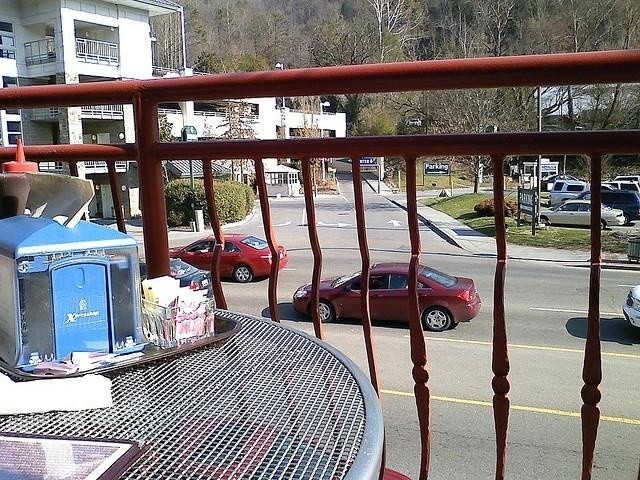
top-left (628, 236), bottom-right (640, 262)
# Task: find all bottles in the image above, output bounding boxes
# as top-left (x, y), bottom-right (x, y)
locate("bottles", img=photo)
top-left (2, 139), bottom-right (38, 173)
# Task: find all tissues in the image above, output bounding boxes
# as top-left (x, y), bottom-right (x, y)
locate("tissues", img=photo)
top-left (0, 174), bottom-right (142, 366)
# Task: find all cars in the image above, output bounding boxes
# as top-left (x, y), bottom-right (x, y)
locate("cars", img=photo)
top-left (540, 174), bottom-right (640, 230)
top-left (292, 262), bottom-right (482, 332)
top-left (140, 233), bottom-right (288, 298)
top-left (622, 284), bottom-right (640, 328)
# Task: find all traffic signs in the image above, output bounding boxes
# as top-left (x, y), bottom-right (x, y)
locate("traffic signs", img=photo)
top-left (423, 162), bottom-right (451, 176)
top-left (517, 188), bottom-right (535, 216)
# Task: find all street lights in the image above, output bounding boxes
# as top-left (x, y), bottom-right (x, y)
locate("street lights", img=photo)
top-left (319, 101), bottom-right (331, 181)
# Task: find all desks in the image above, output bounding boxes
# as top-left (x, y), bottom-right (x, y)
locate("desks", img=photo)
top-left (0, 305), bottom-right (384, 480)
